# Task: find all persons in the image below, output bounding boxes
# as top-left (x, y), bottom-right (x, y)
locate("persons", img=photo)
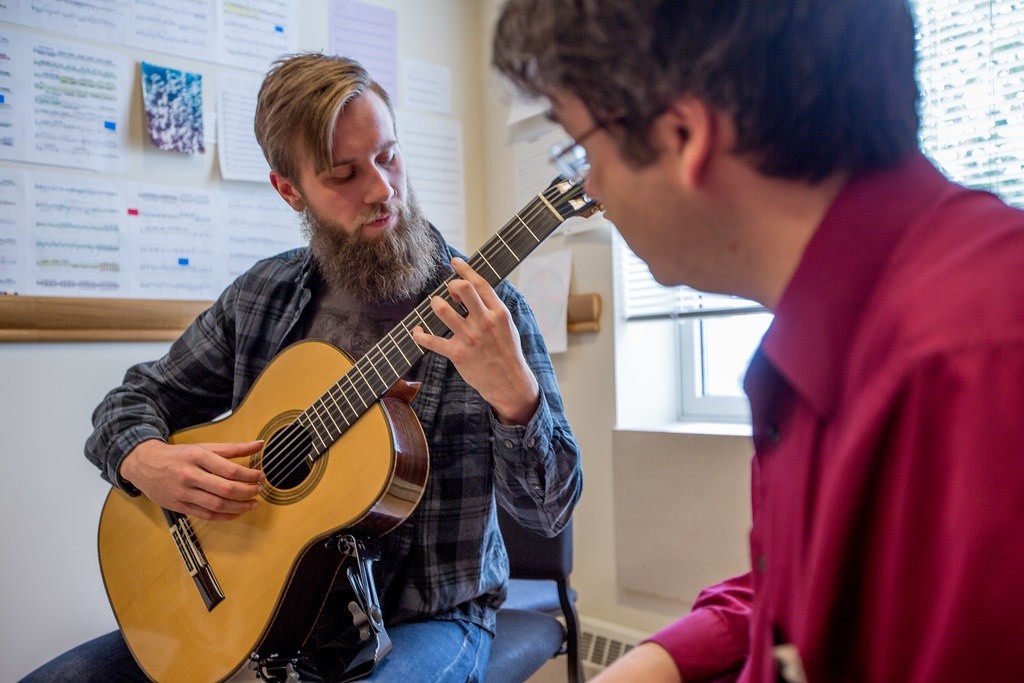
top-left (17, 53), bottom-right (584, 683)
top-left (490, 0), bottom-right (1024, 683)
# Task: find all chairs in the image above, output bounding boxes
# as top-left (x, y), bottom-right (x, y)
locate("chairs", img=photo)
top-left (480, 512), bottom-right (585, 683)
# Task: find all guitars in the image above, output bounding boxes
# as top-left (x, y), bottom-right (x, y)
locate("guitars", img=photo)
top-left (95, 151), bottom-right (606, 683)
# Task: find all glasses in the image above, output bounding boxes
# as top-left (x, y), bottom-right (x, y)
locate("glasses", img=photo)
top-left (549, 120), bottom-right (619, 184)
